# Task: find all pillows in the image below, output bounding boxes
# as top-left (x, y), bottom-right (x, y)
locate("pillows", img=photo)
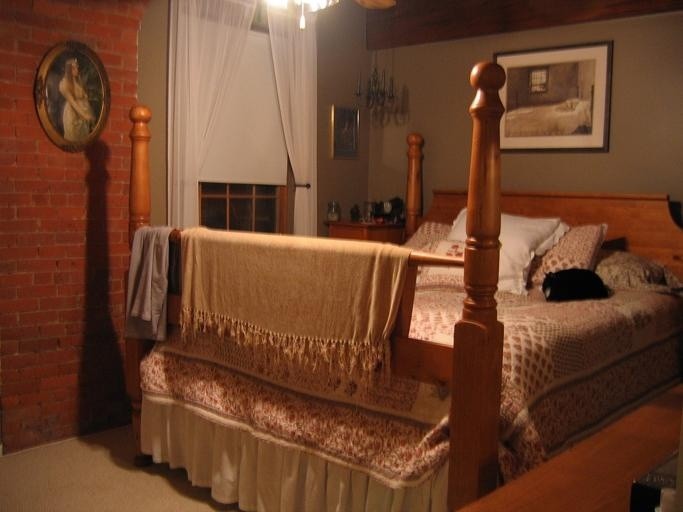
top-left (423, 205), bottom-right (683, 293)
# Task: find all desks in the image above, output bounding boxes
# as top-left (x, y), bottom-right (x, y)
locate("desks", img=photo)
top-left (455, 379), bottom-right (683, 512)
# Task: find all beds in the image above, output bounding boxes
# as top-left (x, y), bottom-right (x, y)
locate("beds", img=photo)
top-left (129, 57), bottom-right (682, 512)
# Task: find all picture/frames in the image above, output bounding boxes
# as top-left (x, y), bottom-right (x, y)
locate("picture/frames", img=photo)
top-left (33, 40), bottom-right (111, 151)
top-left (490, 39), bottom-right (614, 153)
top-left (330, 104), bottom-right (360, 161)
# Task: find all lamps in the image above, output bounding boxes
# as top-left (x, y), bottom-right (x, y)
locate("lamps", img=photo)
top-left (356, 63), bottom-right (396, 106)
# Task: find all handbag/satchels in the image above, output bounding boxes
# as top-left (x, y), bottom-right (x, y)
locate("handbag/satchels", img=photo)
top-left (542, 269), bottom-right (609, 301)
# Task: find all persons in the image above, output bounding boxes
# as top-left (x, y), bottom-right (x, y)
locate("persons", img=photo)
top-left (58, 58), bottom-right (94, 143)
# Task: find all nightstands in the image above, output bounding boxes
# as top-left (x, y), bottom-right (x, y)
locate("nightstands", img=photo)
top-left (323, 218), bottom-right (404, 243)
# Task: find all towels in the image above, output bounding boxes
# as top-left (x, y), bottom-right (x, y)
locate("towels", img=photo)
top-left (124, 225), bottom-right (178, 341)
